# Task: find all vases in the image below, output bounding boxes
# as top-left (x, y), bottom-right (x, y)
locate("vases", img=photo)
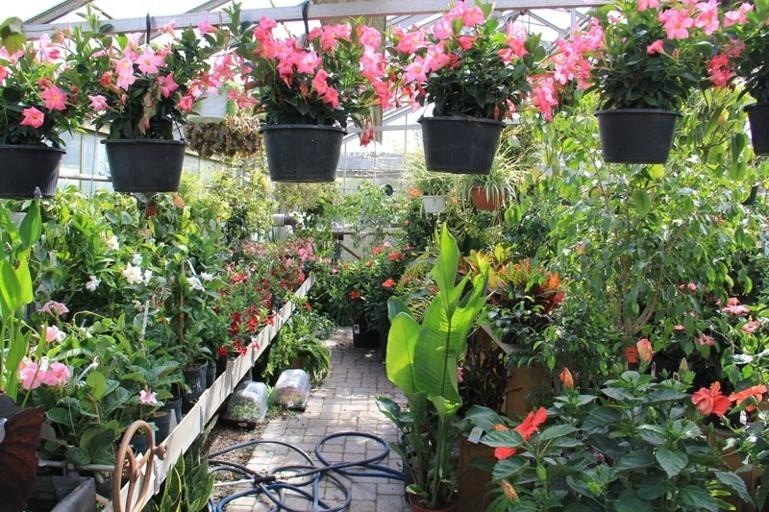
top-left (594, 108), bottom-right (681, 165)
top-left (0, 145), bottom-right (68, 201)
top-left (100, 136), bottom-right (190, 192)
top-left (257, 120), bottom-right (345, 182)
top-left (419, 111), bottom-right (506, 177)
top-left (741, 104), bottom-right (767, 152)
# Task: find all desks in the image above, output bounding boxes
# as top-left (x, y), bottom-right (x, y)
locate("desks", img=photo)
top-left (472, 313), bottom-right (554, 424)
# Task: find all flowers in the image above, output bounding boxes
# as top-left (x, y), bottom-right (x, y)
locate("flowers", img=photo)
top-left (85, 0), bottom-right (255, 141)
top-left (0, 14), bottom-right (112, 145)
top-left (707, 0), bottom-right (767, 101)
top-left (0, 227), bottom-right (330, 512)
top-left (385, 0), bottom-right (555, 127)
top-left (229, 2), bottom-right (394, 146)
top-left (547, 1), bottom-right (707, 112)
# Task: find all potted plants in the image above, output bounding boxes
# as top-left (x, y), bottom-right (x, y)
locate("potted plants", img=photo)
top-left (455, 330), bottom-right (524, 494)
top-left (399, 137), bottom-right (461, 215)
top-left (379, 218), bottom-right (501, 511)
top-left (452, 128), bottom-right (534, 214)
